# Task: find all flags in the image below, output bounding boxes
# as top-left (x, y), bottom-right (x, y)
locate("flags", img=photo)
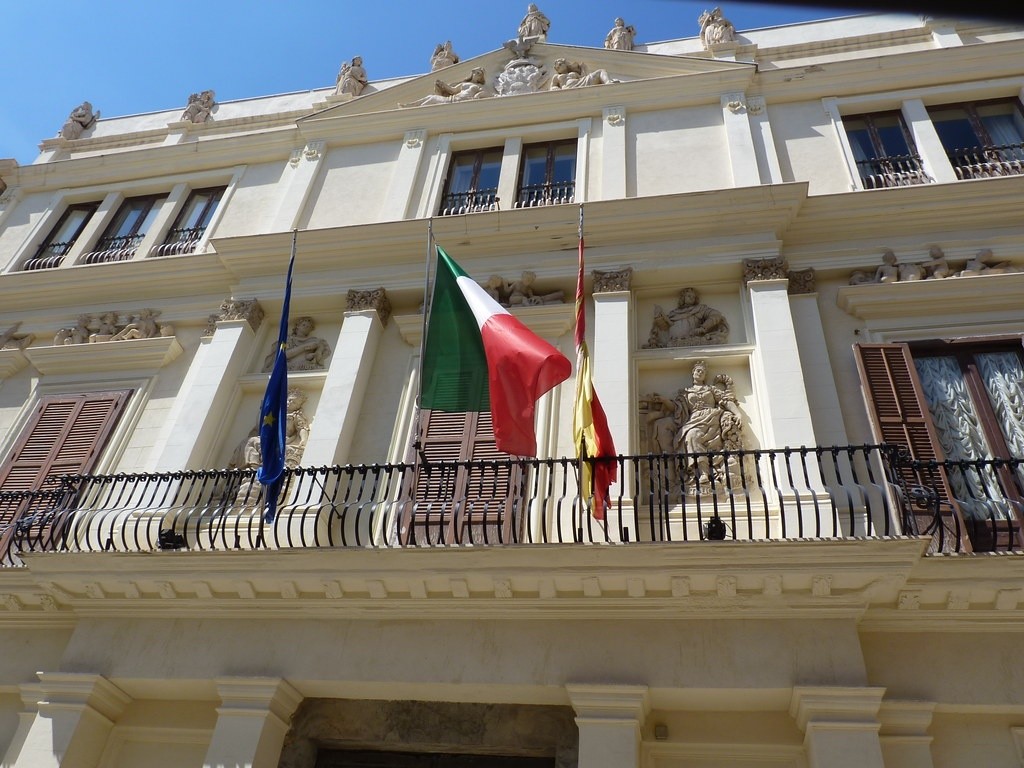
top-left (260, 259), bottom-right (293, 517)
top-left (572, 238), bottom-right (615, 520)
top-left (422, 243), bottom-right (571, 457)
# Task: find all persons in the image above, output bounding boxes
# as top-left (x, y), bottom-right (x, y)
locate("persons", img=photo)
top-left (654, 362), bottom-right (743, 482)
top-left (503, 272), bottom-right (540, 307)
top-left (334, 56), bottom-right (366, 95)
top-left (183, 91), bottom-right (211, 122)
top-left (478, 275), bottom-right (502, 302)
top-left (606, 18), bottom-right (635, 50)
top-left (700, 10), bottom-right (735, 44)
top-left (520, 4), bottom-right (549, 36)
top-left (62, 101), bottom-right (93, 139)
top-left (432, 41), bottom-right (456, 71)
top-left (660, 288), bottom-right (722, 345)
top-left (551, 59), bottom-right (621, 91)
top-left (398, 68), bottom-right (486, 109)
top-left (272, 316), bottom-right (329, 368)
top-left (245, 393), bottom-right (309, 467)
top-left (872, 246), bottom-right (992, 283)
top-left (63, 308), bottom-right (161, 344)
